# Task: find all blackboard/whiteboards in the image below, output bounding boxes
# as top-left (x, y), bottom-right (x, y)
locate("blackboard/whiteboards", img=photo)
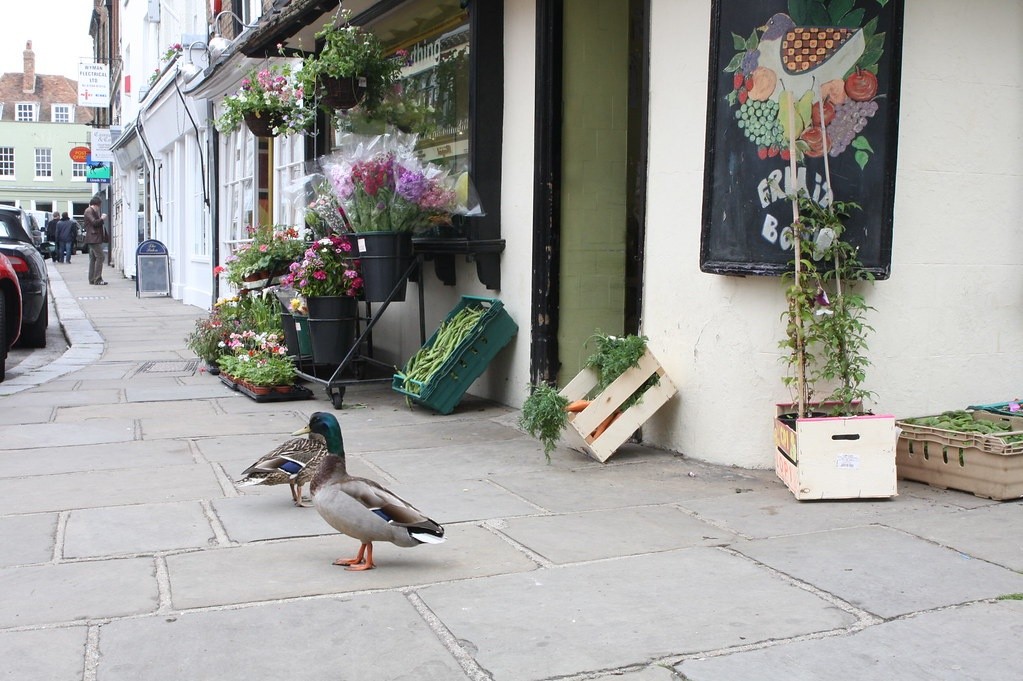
top-left (137, 255), bottom-right (169, 294)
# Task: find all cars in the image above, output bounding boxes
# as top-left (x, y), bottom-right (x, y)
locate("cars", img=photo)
top-left (71, 220), bottom-right (88, 255)
top-left (0, 252), bottom-right (21, 384)
top-left (0, 212), bottom-right (49, 349)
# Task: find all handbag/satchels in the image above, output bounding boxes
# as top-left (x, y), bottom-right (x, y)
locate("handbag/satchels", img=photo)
top-left (102, 225), bottom-right (109, 243)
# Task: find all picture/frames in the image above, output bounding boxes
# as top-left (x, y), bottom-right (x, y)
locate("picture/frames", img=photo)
top-left (698, 0), bottom-right (905, 281)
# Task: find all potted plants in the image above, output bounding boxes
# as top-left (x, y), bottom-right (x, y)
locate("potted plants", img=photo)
top-left (897, 410), bottom-right (1023, 501)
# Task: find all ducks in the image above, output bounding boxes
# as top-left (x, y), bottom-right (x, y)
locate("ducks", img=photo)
top-left (233, 434), bottom-right (327, 508)
top-left (294, 408), bottom-right (448, 572)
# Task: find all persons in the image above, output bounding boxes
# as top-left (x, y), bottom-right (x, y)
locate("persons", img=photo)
top-left (84, 197), bottom-right (108, 285)
top-left (55, 212), bottom-right (77, 264)
top-left (47, 211), bottom-right (61, 263)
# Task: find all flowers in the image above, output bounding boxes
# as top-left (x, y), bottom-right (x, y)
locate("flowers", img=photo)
top-left (1003, 400), bottom-right (1023, 415)
top-left (184, 8), bottom-right (460, 385)
top-left (777, 187), bottom-right (879, 420)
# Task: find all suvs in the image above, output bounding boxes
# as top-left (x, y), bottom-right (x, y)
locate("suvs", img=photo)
top-left (0, 204), bottom-right (56, 257)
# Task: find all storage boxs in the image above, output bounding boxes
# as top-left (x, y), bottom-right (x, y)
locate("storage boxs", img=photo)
top-left (392, 294), bottom-right (520, 414)
top-left (558, 336), bottom-right (678, 462)
top-left (775, 400), bottom-right (898, 502)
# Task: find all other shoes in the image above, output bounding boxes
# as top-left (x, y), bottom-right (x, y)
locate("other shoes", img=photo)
top-left (89, 280), bottom-right (94, 284)
top-left (59, 259), bottom-right (64, 263)
top-left (66, 260), bottom-right (71, 263)
top-left (94, 278), bottom-right (108, 285)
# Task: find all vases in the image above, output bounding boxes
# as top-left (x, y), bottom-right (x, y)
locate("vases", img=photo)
top-left (242, 107), bottom-right (291, 137)
top-left (281, 313), bottom-right (299, 356)
top-left (245, 273), bottom-right (259, 282)
top-left (830, 411), bottom-right (875, 439)
top-left (313, 72), bottom-right (368, 110)
top-left (276, 269), bottom-right (285, 276)
top-left (777, 412), bottom-right (827, 430)
top-left (222, 372), bottom-right (291, 395)
top-left (292, 315), bottom-right (313, 357)
top-left (306, 296), bottom-right (358, 363)
top-left (978, 399), bottom-right (1023, 417)
top-left (355, 231), bottom-right (412, 301)
top-left (241, 289), bottom-right (250, 295)
top-left (339, 232), bottom-right (360, 262)
top-left (259, 269), bottom-right (269, 278)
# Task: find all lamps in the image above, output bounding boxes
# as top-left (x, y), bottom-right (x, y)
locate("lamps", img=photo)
top-left (208, 11), bottom-right (259, 64)
top-left (183, 41), bottom-right (210, 84)
